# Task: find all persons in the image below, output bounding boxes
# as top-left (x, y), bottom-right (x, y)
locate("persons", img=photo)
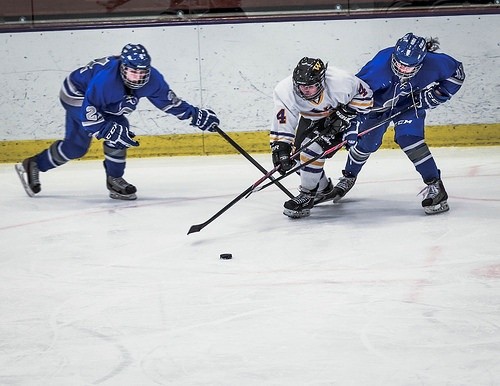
top-left (15, 42), bottom-right (220, 200)
top-left (269, 54), bottom-right (374, 218)
top-left (331, 34), bottom-right (465, 213)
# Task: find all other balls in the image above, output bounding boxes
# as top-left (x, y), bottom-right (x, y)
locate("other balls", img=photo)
top-left (219, 253), bottom-right (233, 260)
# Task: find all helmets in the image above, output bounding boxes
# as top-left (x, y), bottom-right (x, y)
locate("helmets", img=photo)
top-left (293, 57), bottom-right (325, 86)
top-left (121, 44), bottom-right (152, 71)
top-left (393, 32), bottom-right (427, 66)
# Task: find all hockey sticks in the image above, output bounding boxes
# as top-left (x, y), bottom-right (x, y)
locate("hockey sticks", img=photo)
top-left (244, 101), bottom-right (420, 200)
top-left (186, 124), bottom-right (333, 236)
top-left (213, 124), bottom-right (296, 199)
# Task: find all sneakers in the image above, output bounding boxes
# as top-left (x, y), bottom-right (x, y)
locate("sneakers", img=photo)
top-left (284, 183), bottom-right (320, 219)
top-left (15, 149), bottom-right (47, 197)
top-left (314, 177), bottom-right (337, 205)
top-left (333, 169), bottom-right (356, 202)
top-left (417, 169), bottom-right (450, 214)
top-left (104, 160), bottom-right (137, 200)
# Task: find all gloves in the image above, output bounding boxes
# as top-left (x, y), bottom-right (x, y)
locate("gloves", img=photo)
top-left (98, 121), bottom-right (139, 150)
top-left (190, 107), bottom-right (220, 132)
top-left (270, 141), bottom-right (296, 175)
top-left (342, 121), bottom-right (360, 150)
top-left (325, 105), bottom-right (358, 135)
top-left (412, 81), bottom-right (450, 109)
